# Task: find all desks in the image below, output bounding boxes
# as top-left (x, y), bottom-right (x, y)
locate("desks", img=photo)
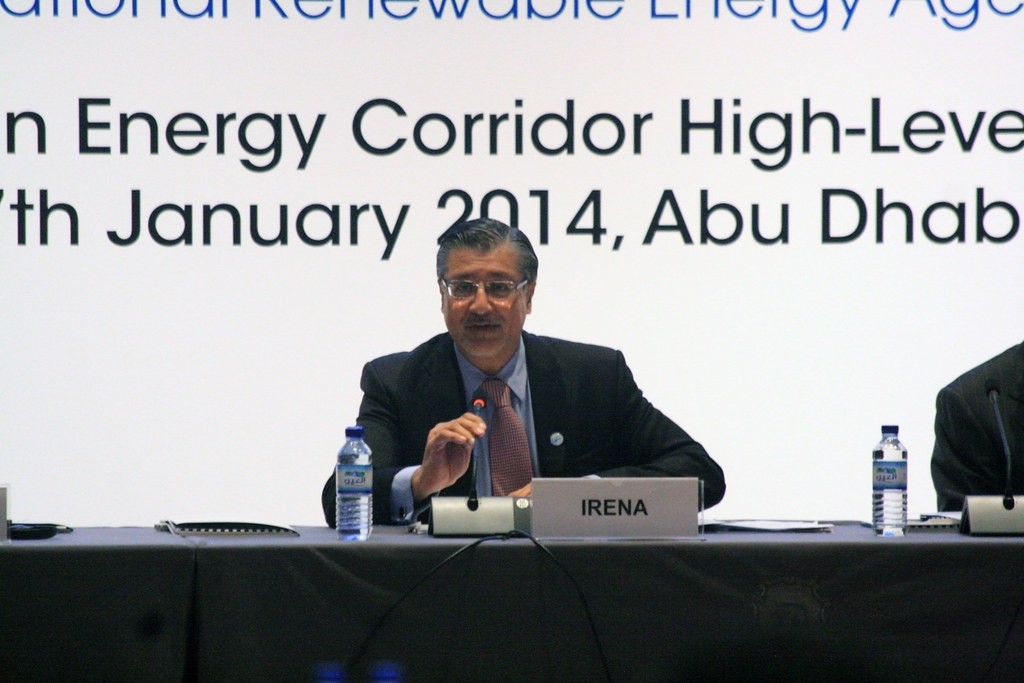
top-left (176, 520), bottom-right (1024, 683)
top-left (0, 526), bottom-right (197, 682)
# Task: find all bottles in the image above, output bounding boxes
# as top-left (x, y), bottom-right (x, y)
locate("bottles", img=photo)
top-left (335, 425), bottom-right (371, 542)
top-left (872, 425), bottom-right (907, 537)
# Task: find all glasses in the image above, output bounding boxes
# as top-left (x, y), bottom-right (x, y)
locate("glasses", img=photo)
top-left (441, 278), bottom-right (527, 300)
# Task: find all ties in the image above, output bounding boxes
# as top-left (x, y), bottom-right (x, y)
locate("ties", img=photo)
top-left (479, 379), bottom-right (532, 496)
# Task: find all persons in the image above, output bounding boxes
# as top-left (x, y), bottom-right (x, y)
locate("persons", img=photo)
top-left (321, 217), bottom-right (725, 527)
top-left (930, 340), bottom-right (1024, 513)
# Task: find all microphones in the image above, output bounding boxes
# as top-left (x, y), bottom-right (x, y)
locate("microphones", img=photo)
top-left (958, 378), bottom-right (1024, 536)
top-left (427, 395), bottom-right (532, 539)
